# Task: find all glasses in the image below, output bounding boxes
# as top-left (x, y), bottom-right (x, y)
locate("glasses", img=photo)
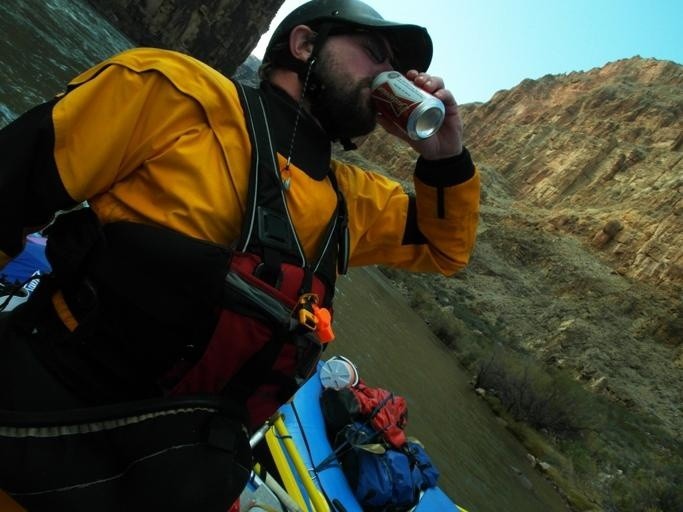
top-left (348, 26), bottom-right (395, 64)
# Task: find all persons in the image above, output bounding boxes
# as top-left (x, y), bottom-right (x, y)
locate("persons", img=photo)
top-left (1, 4), bottom-right (479, 512)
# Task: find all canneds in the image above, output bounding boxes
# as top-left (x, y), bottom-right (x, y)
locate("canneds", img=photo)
top-left (371, 70), bottom-right (446, 142)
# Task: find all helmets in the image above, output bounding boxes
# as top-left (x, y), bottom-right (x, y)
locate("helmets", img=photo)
top-left (264, 2), bottom-right (432, 74)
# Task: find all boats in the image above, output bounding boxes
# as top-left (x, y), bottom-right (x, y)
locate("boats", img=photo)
top-left (259, 354), bottom-right (474, 512)
top-left (0, 230), bottom-right (283, 512)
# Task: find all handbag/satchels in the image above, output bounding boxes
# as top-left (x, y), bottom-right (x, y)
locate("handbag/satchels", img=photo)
top-left (320, 378), bottom-right (439, 512)
